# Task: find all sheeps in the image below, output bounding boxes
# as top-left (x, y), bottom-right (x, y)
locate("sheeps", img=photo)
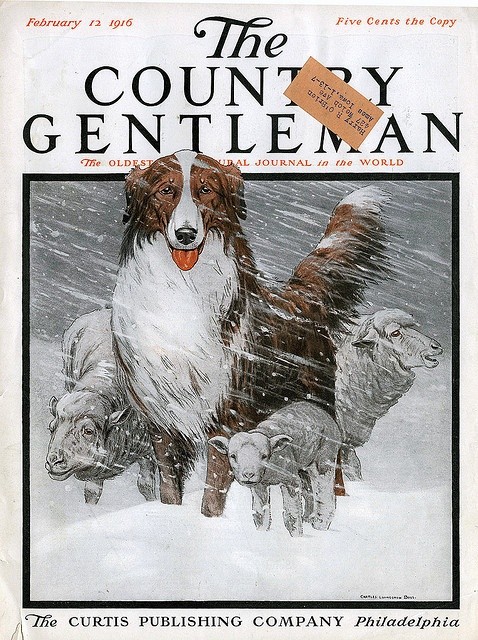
top-left (208, 402), bottom-right (343, 539)
top-left (43, 307), bottom-right (158, 507)
top-left (334, 309), bottom-right (443, 482)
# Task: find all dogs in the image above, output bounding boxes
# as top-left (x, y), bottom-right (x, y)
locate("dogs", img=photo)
top-left (109, 149), bottom-right (403, 520)
